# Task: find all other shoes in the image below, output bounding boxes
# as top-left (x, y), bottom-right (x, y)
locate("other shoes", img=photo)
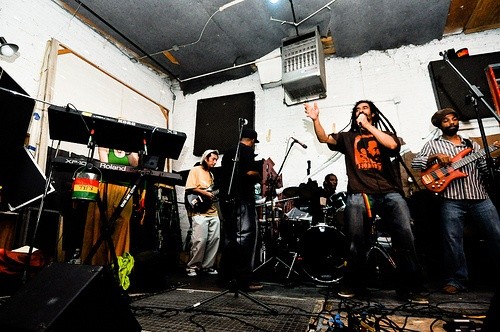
top-left (396, 288), bottom-right (430, 304)
top-left (201, 266), bottom-right (219, 275)
top-left (442, 284), bottom-right (461, 294)
top-left (338, 285), bottom-right (376, 298)
top-left (184, 267), bottom-right (198, 277)
top-left (239, 283), bottom-right (264, 292)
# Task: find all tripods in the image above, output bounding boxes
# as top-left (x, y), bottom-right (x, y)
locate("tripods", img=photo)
top-left (184, 122), bottom-right (301, 314)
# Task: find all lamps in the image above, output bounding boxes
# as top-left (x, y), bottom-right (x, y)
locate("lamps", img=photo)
top-left (0, 37), bottom-right (19, 57)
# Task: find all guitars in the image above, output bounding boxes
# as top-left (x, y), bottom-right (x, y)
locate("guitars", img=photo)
top-left (420, 143), bottom-right (500, 192)
top-left (185, 188), bottom-right (220, 214)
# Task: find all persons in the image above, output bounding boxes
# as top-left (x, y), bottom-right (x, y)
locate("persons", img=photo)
top-left (80, 147), bottom-right (140, 266)
top-left (317, 174), bottom-right (337, 223)
top-left (185, 150), bottom-right (220, 276)
top-left (303, 100), bottom-right (429, 304)
top-left (219, 129), bottom-right (263, 288)
top-left (411, 108), bottom-right (500, 294)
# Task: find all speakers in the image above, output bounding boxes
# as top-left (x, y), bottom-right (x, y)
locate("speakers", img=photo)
top-left (0, 261), bottom-right (143, 332)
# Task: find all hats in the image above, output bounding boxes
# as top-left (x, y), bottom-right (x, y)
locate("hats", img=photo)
top-left (431, 108), bottom-right (458, 127)
top-left (200, 149), bottom-right (219, 165)
top-left (241, 129), bottom-right (261, 143)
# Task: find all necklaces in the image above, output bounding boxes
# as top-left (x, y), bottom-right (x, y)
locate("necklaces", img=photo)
top-left (117, 149), bottom-right (122, 153)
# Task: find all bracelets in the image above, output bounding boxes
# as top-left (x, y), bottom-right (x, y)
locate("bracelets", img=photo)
top-left (125, 151), bottom-right (132, 155)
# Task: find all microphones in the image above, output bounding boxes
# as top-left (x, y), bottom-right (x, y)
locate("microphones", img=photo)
top-left (359, 123), bottom-right (362, 129)
top-left (292, 137), bottom-right (307, 149)
top-left (239, 118), bottom-right (248, 125)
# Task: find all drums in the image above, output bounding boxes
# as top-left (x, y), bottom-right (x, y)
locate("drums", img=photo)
top-left (257, 216), bottom-right (350, 284)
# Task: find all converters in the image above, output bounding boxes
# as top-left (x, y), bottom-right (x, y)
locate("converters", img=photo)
top-left (448, 319), bottom-right (476, 330)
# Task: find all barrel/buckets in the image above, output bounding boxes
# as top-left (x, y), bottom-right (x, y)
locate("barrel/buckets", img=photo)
top-left (71, 164), bottom-right (102, 202)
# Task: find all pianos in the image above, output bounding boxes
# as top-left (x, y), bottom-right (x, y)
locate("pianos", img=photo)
top-left (47, 102), bottom-right (187, 160)
top-left (51, 157), bottom-right (183, 263)
